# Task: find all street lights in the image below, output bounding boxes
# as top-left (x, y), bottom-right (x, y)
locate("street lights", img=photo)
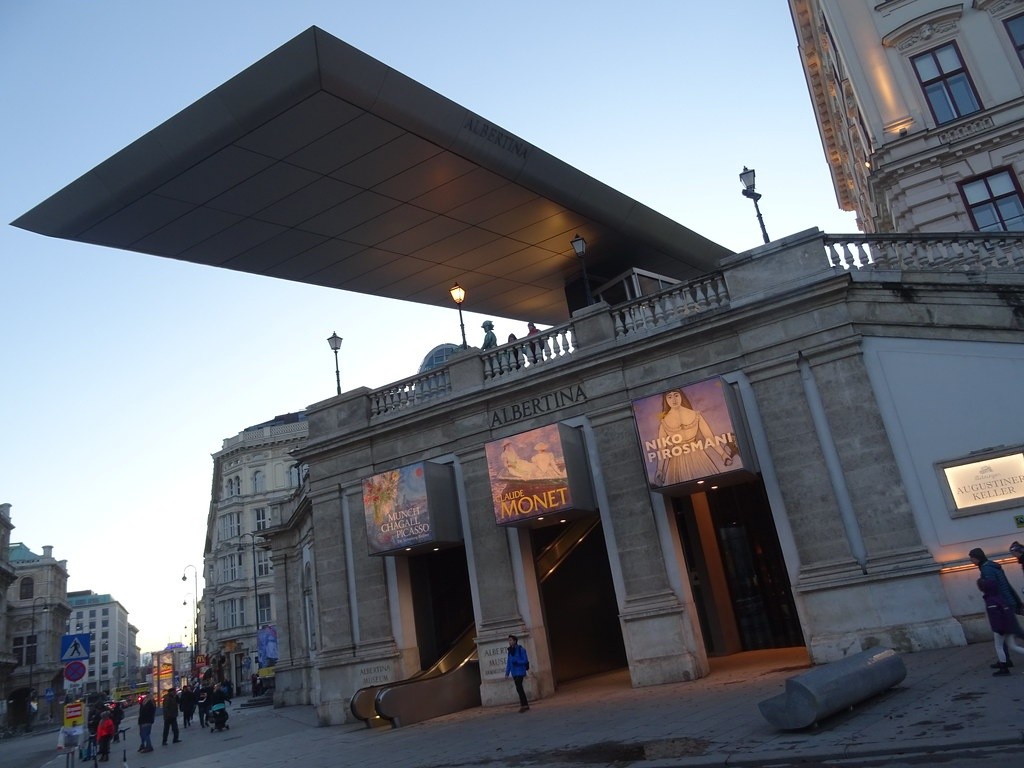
top-left (449, 281), bottom-right (468, 351)
top-left (182, 565), bottom-right (199, 675)
top-left (570, 233), bottom-right (594, 306)
top-left (327, 330), bottom-right (343, 395)
top-left (26, 597), bottom-right (49, 731)
top-left (238, 533), bottom-right (260, 631)
top-left (739, 166), bottom-right (772, 244)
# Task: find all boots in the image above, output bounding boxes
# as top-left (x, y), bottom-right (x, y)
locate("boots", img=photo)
top-left (993, 662), bottom-right (1009, 675)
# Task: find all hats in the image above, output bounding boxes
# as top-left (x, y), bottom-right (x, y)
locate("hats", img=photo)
top-left (101, 711), bottom-right (110, 720)
top-left (969, 548), bottom-right (985, 557)
top-left (528, 321), bottom-right (534, 326)
top-left (481, 321), bottom-right (492, 328)
top-left (1009, 541), bottom-right (1022, 551)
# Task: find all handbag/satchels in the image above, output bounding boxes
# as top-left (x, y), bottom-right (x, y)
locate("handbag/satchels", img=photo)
top-left (982, 566), bottom-right (1015, 632)
top-left (198, 693), bottom-right (207, 702)
top-left (180, 704), bottom-right (183, 711)
top-left (224, 687), bottom-right (228, 693)
top-left (519, 645), bottom-right (530, 671)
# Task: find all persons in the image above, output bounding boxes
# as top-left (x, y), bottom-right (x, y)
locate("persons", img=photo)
top-left (252, 674), bottom-right (262, 697)
top-left (527, 321), bottom-right (544, 362)
top-left (481, 321), bottom-right (497, 350)
top-left (180, 683), bottom-right (231, 728)
top-left (507, 334), bottom-right (523, 371)
top-left (137, 694), bottom-right (155, 753)
top-left (505, 635), bottom-right (529, 713)
top-left (969, 540), bottom-right (1024, 677)
top-left (82, 700), bottom-right (125, 762)
top-left (161, 688), bottom-right (182, 745)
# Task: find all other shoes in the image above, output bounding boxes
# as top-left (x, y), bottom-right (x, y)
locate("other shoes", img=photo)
top-left (144, 746), bottom-right (153, 752)
top-left (173, 739), bottom-right (182, 743)
top-left (519, 707), bottom-right (529, 712)
top-left (991, 660), bottom-right (1013, 667)
top-left (162, 742), bottom-right (168, 745)
top-left (137, 747), bottom-right (145, 752)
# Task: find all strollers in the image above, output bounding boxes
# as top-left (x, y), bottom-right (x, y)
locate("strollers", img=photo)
top-left (209, 703), bottom-right (230, 732)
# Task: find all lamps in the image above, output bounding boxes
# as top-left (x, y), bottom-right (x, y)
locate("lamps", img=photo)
top-left (900, 128), bottom-right (907, 136)
